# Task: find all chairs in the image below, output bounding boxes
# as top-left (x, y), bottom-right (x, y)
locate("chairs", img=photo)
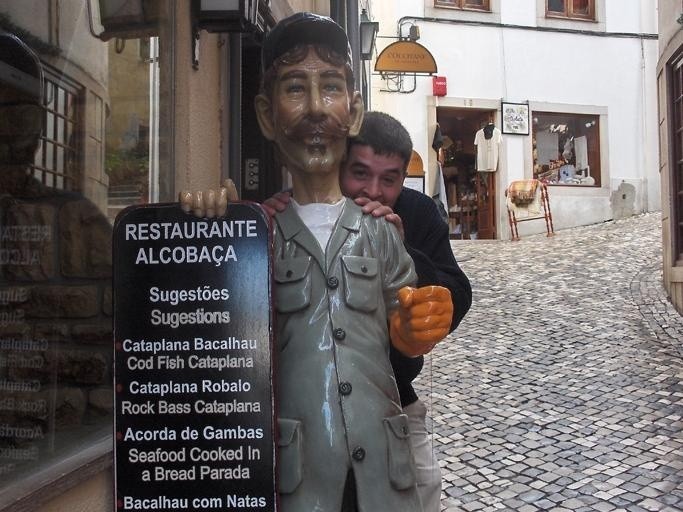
top-left (504, 178), bottom-right (557, 242)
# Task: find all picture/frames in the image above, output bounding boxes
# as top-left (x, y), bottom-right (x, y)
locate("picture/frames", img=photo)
top-left (500, 100), bottom-right (530, 136)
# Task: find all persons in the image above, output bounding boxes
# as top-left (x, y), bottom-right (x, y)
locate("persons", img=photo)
top-left (177, 11), bottom-right (456, 512)
top-left (253, 110), bottom-right (472, 512)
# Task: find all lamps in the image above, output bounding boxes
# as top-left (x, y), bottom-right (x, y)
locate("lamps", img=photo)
top-left (362, 8), bottom-right (378, 61)
